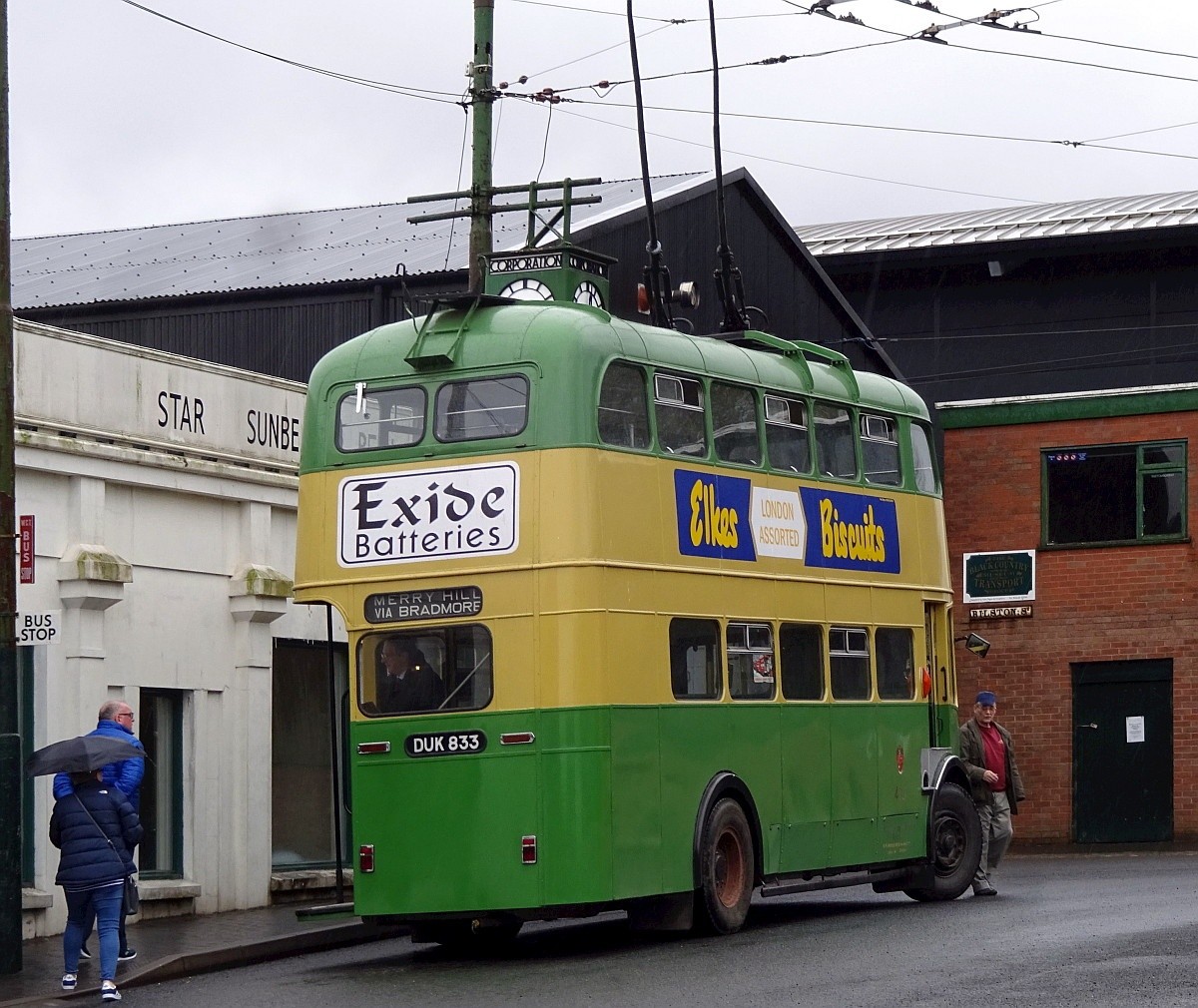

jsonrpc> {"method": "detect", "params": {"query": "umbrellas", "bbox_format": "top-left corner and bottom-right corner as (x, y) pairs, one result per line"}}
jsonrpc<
(27, 737), (146, 777)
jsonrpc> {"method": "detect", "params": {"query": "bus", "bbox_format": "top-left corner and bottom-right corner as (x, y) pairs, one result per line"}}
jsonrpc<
(292, 178), (994, 952)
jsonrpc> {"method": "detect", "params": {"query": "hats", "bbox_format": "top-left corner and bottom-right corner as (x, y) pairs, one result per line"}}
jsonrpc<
(976, 691), (996, 706)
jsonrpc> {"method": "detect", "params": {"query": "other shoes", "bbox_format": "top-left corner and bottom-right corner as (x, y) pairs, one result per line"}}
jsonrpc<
(975, 888), (997, 896)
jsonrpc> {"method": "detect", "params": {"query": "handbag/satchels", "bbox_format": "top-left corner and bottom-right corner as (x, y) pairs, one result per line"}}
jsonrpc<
(123, 875), (139, 916)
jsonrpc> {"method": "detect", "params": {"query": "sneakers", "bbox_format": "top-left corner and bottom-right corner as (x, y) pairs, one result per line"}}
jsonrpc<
(117, 948), (137, 961)
(62, 974), (78, 990)
(79, 946), (91, 958)
(101, 985), (122, 1000)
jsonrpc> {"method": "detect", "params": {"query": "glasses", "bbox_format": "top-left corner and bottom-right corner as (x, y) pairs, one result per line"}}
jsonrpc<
(113, 712), (134, 720)
(380, 652), (400, 659)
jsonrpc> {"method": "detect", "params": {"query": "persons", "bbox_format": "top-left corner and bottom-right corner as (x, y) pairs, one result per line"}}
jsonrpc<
(959, 692), (1026, 896)
(49, 770), (144, 999)
(377, 636), (445, 714)
(52, 700), (145, 961)
